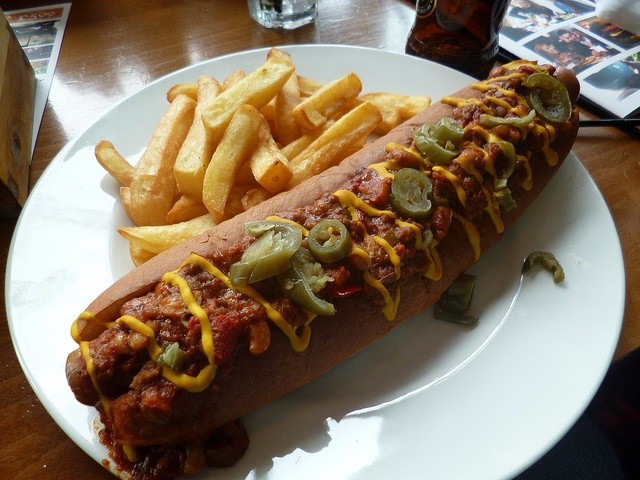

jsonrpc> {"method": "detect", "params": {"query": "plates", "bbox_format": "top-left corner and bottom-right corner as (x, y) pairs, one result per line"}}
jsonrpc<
(4, 43), (627, 480)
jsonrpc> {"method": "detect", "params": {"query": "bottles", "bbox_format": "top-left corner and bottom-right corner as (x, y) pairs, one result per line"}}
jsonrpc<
(405, 0), (512, 85)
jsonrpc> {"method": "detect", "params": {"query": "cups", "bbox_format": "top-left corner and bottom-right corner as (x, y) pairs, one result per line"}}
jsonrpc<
(248, 0), (319, 31)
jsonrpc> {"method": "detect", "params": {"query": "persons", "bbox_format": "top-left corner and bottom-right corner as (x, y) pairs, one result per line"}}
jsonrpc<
(533, 42), (581, 68)
(499, 18), (534, 43)
(558, 30), (620, 57)
(509, 0), (561, 25)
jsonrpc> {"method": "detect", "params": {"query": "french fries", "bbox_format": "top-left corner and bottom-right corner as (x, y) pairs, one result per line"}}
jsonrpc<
(93, 52), (426, 266)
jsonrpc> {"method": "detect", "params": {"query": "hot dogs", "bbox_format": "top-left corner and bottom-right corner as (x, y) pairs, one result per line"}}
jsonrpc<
(63, 57), (581, 480)
